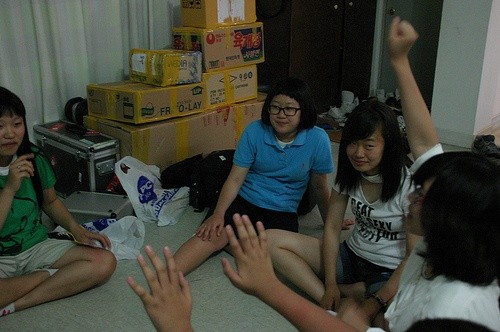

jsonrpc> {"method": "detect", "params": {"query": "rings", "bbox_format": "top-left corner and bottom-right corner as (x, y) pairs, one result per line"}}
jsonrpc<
(17, 165), (21, 168)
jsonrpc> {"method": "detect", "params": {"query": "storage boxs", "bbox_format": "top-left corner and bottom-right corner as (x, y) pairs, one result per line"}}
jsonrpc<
(62, 190), (133, 225)
(172, 22), (265, 73)
(87, 81), (207, 126)
(83, 95), (266, 170)
(180, 0), (256, 29)
(203, 65), (258, 111)
(128, 48), (202, 87)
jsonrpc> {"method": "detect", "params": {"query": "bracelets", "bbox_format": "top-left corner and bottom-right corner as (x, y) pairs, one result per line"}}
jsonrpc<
(373, 293), (388, 308)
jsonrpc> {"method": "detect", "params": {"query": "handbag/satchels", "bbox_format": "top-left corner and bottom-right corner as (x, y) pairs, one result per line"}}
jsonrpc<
(80, 216), (147, 259)
(195, 148), (236, 213)
(114, 158), (190, 227)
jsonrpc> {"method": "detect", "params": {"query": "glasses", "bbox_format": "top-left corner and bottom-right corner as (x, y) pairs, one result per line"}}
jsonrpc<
(413, 185), (425, 203)
(267, 103), (302, 116)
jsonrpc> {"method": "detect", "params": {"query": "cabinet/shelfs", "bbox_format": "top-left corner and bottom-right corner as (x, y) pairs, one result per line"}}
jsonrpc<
(256, 0), (376, 112)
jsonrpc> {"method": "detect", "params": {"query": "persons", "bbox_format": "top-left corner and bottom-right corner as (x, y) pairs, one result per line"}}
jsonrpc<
(173, 83), (355, 278)
(127, 213), (494, 332)
(338, 16), (500, 332)
(265, 98), (422, 324)
(0, 86), (117, 318)
